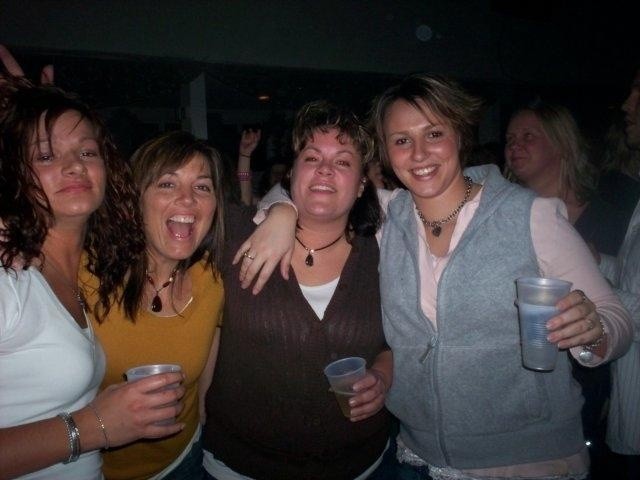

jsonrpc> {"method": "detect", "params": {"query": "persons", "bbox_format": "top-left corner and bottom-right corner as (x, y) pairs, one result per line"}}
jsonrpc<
(2, 42), (639, 480)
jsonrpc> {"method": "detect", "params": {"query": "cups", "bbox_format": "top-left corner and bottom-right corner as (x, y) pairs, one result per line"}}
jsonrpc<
(512, 277), (574, 373)
(125, 364), (182, 426)
(323, 356), (367, 419)
